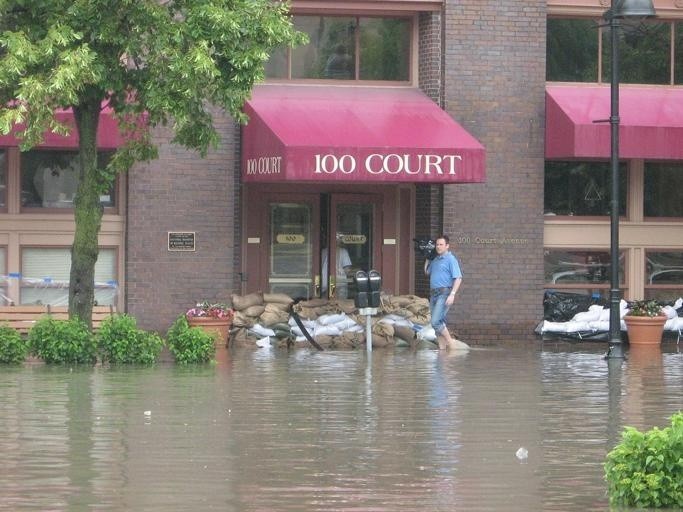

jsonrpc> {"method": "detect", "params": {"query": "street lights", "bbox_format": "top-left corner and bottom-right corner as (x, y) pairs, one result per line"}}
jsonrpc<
(605, 0), (658, 360)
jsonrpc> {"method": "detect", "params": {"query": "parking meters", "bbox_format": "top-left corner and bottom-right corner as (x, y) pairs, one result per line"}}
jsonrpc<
(353, 270), (382, 352)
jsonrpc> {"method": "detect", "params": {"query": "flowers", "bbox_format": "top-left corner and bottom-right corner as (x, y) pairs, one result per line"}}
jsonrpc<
(186, 298), (234, 323)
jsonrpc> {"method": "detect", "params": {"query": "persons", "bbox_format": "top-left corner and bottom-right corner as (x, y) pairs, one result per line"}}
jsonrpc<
(424, 235), (464, 349)
(318, 245), (353, 300)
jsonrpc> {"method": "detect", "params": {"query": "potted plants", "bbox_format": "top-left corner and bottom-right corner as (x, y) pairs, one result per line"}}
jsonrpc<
(622, 298), (668, 345)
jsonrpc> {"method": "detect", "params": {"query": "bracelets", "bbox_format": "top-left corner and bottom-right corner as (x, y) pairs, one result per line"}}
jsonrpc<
(450, 291), (457, 296)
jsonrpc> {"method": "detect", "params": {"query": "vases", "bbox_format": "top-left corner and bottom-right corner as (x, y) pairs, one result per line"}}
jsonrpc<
(187, 317), (233, 349)
